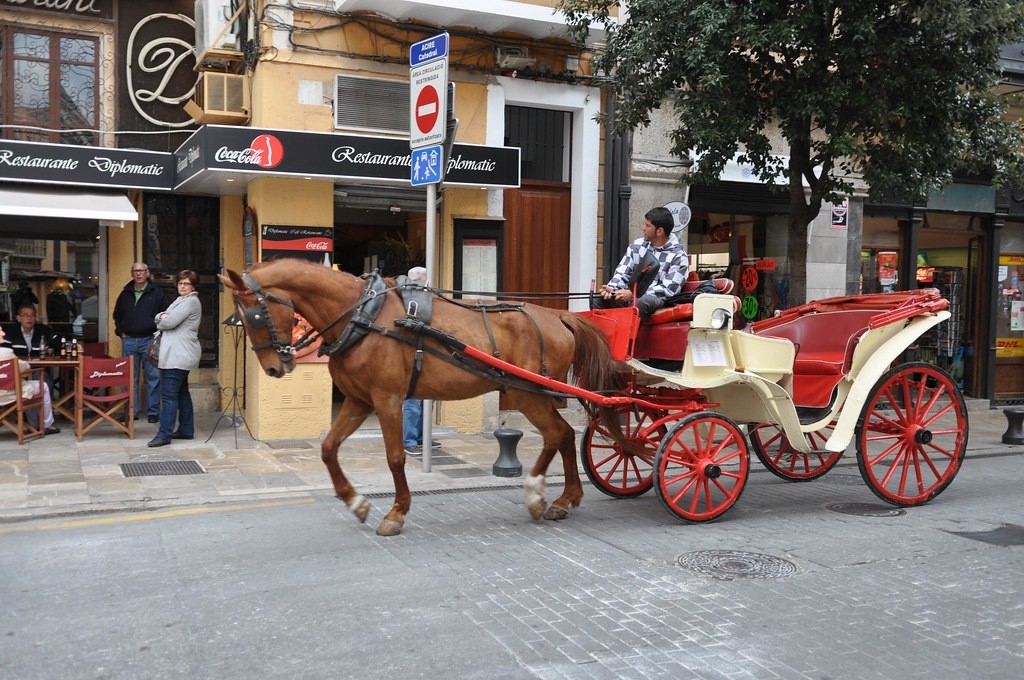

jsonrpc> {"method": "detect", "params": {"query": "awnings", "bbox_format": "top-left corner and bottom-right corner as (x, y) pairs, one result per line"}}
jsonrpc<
(0, 183), (138, 222)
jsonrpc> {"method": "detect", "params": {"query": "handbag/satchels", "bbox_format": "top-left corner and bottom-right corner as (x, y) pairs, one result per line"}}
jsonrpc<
(145, 331), (162, 360)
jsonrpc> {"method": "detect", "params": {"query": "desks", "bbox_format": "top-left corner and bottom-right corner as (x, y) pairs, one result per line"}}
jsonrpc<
(15, 355), (90, 437)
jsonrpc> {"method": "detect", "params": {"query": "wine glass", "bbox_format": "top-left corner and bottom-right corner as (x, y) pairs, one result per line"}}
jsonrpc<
(67, 350), (71, 358)
(47, 349), (54, 357)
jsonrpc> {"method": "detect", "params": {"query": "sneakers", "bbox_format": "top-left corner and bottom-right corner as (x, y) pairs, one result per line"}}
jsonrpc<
(418, 441), (441, 447)
(405, 446), (422, 455)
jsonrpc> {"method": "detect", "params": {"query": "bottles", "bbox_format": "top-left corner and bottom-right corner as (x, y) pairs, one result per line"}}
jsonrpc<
(61, 336), (66, 359)
(40, 336), (45, 360)
(88, 272), (99, 286)
(70, 271), (84, 286)
(71, 336), (78, 359)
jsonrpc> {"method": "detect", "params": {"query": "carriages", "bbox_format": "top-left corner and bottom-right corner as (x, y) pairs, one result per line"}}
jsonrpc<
(215, 257), (971, 536)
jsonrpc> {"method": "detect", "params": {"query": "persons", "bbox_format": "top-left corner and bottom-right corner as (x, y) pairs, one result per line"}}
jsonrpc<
(113, 263), (168, 422)
(593, 207), (689, 318)
(0, 326), (61, 436)
(403, 267), (442, 455)
(47, 286), (77, 322)
(4, 302), (60, 389)
(148, 270), (202, 447)
(11, 281), (39, 321)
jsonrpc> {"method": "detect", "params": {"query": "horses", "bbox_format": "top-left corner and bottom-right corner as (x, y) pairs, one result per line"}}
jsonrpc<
(216, 261), (634, 538)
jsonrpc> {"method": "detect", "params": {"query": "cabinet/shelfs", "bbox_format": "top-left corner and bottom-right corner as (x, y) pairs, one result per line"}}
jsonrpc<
(914, 266), (965, 358)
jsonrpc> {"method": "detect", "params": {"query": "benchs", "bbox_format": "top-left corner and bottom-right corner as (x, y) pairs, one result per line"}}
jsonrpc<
(756, 309), (893, 408)
(633, 278), (735, 360)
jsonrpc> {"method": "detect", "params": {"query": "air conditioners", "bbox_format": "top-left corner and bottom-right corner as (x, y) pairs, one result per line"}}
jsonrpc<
(195, 0), (241, 69)
(194, 71), (248, 124)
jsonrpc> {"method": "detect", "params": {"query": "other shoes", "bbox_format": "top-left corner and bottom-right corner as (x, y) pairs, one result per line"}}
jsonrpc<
(116, 415), (138, 422)
(148, 416), (158, 422)
(44, 427), (60, 435)
(148, 436), (171, 447)
(171, 431), (193, 439)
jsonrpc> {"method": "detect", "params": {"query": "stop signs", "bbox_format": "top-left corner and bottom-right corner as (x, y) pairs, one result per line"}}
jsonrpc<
(408, 58), (445, 148)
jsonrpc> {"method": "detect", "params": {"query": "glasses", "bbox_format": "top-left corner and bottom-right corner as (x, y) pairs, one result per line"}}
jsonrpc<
(132, 270), (147, 273)
(178, 282), (191, 286)
(19, 313), (36, 318)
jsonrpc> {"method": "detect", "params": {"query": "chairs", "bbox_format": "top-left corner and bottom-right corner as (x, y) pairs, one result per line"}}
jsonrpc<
(0, 342), (135, 442)
